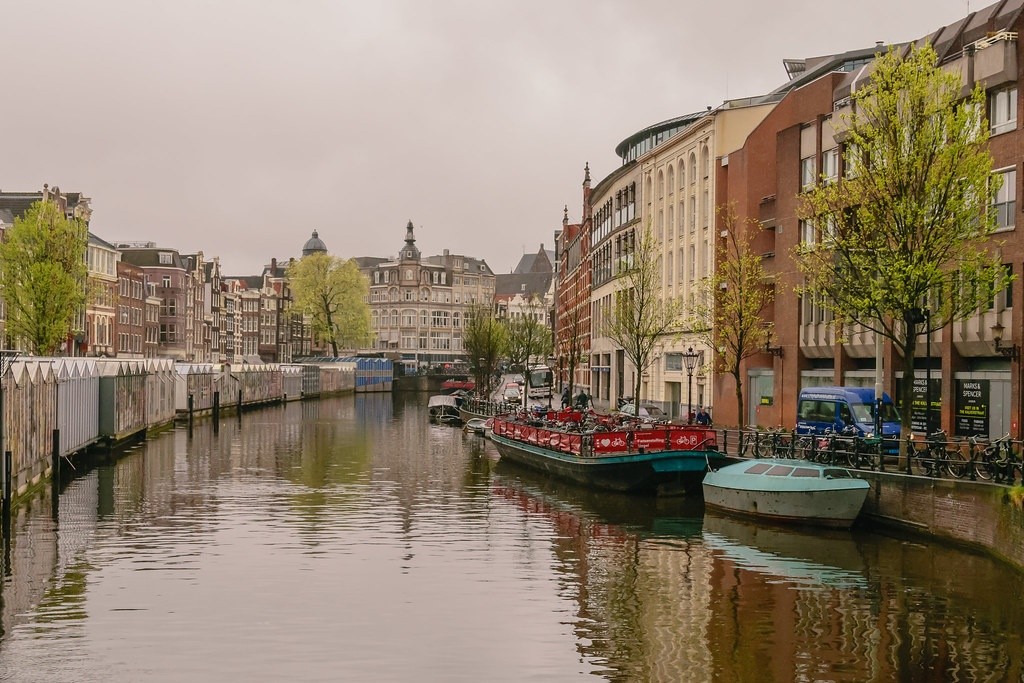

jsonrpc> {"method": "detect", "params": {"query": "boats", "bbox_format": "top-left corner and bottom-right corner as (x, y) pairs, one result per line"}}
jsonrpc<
(428, 389), (466, 423)
(467, 417), (487, 432)
(702, 457), (880, 531)
(481, 417), (494, 438)
(490, 406), (728, 492)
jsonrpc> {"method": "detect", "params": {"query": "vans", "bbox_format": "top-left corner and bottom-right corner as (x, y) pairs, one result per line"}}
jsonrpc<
(796, 387), (915, 461)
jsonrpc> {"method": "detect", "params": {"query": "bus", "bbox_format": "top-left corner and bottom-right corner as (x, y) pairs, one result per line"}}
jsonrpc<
(523, 365), (556, 399)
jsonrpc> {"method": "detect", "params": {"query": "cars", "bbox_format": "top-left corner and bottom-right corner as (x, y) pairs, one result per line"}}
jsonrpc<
(617, 404), (669, 421)
(502, 382), (523, 406)
(512, 374), (526, 385)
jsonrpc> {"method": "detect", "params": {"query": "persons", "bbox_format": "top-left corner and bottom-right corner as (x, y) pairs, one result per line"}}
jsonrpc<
(617, 395), (624, 410)
(560, 388), (569, 409)
(575, 390), (589, 408)
(695, 407), (713, 425)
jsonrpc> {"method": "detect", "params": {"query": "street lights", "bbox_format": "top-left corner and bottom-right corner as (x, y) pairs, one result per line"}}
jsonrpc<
(682, 345), (699, 426)
(905, 308), (931, 437)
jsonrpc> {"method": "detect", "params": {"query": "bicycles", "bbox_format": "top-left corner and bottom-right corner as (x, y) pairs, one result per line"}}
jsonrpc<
(740, 424), (1024, 486)
(512, 402), (662, 452)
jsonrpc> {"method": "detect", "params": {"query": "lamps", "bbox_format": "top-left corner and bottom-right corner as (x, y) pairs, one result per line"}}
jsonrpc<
(989, 322), (1017, 362)
(764, 332), (783, 360)
